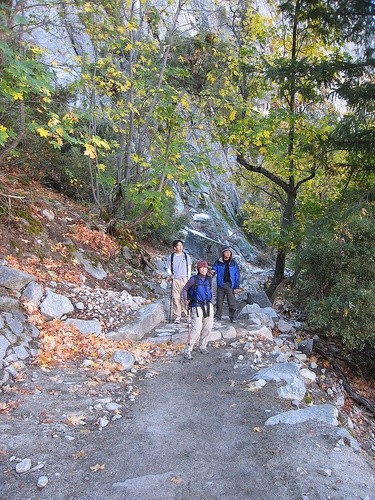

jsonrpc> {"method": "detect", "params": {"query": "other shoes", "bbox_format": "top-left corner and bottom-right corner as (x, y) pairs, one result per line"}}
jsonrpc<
(184, 318), (191, 323)
(184, 353), (193, 360)
(174, 318), (181, 323)
(230, 318), (236, 323)
(198, 349), (209, 354)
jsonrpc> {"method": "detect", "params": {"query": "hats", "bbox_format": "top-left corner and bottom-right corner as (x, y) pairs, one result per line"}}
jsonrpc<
(197, 260), (209, 270)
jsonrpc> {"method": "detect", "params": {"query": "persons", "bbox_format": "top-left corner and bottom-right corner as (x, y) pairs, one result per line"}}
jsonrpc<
(166, 240), (193, 324)
(182, 261), (214, 360)
(208, 246), (240, 323)
